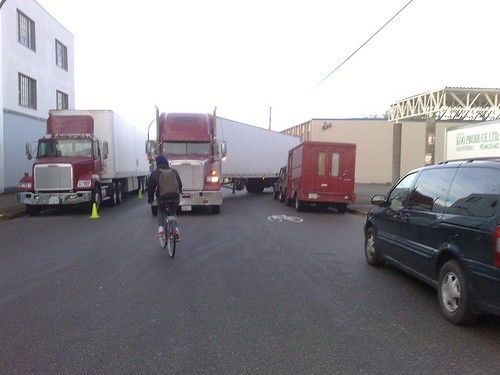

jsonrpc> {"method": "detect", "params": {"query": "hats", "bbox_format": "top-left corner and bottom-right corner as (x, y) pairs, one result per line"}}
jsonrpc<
(157, 156), (168, 166)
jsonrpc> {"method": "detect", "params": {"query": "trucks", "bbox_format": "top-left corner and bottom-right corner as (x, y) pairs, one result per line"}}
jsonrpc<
(284, 140), (357, 212)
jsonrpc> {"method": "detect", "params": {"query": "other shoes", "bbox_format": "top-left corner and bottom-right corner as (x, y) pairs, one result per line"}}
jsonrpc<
(157, 232), (164, 237)
(171, 229), (177, 234)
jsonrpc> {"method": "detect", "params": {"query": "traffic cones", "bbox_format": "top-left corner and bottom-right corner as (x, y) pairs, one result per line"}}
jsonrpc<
(138, 189), (143, 199)
(89, 202), (100, 219)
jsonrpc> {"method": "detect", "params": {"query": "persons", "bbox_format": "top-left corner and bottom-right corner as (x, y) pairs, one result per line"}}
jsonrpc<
(148, 155), (183, 242)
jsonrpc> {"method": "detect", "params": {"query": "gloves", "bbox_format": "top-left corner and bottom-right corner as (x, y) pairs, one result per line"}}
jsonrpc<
(148, 199), (154, 204)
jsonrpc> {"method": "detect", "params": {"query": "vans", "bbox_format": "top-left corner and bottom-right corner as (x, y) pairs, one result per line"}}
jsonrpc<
(363, 158), (500, 325)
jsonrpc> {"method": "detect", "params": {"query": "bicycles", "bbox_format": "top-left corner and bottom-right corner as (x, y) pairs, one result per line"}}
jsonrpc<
(150, 200), (178, 258)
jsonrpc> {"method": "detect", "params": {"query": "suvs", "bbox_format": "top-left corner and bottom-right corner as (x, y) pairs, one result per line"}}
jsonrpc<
(273, 166), (287, 203)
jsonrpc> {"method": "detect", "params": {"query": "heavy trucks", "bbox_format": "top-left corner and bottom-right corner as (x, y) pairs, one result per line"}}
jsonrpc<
(145, 104), (303, 215)
(15, 108), (152, 215)
